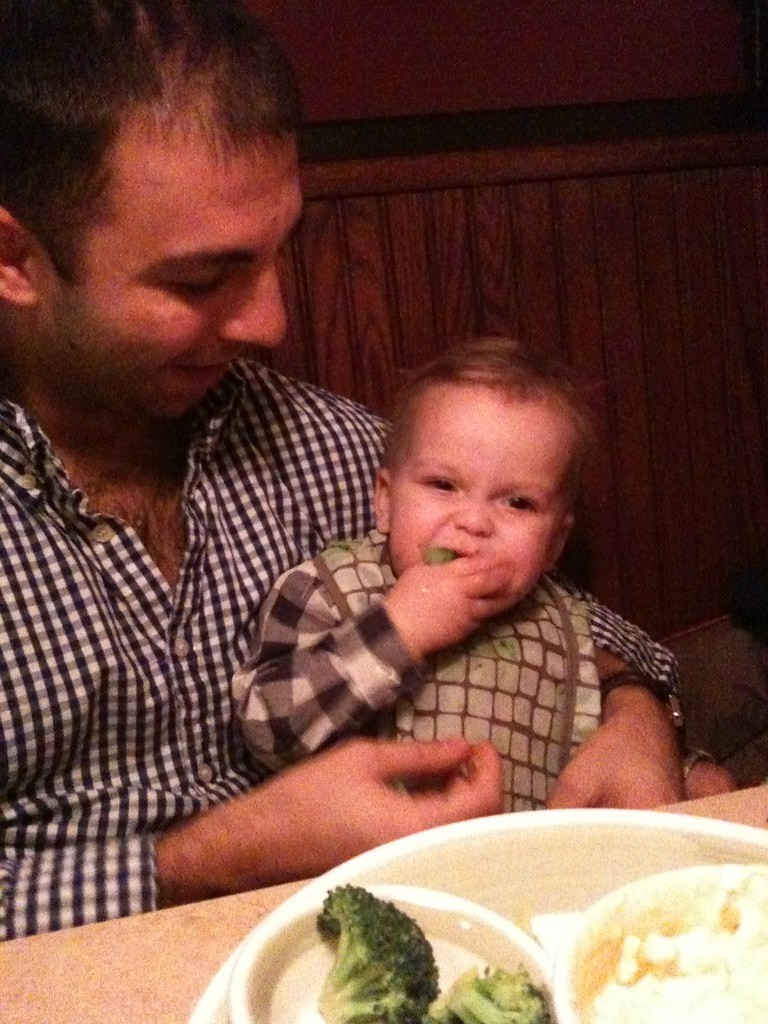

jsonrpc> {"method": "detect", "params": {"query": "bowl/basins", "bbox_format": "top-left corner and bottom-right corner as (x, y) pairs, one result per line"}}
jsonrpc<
(228, 885), (563, 1024)
(554, 863), (768, 1024)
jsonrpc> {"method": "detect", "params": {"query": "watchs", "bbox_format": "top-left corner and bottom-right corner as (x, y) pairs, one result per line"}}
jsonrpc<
(600, 670), (687, 728)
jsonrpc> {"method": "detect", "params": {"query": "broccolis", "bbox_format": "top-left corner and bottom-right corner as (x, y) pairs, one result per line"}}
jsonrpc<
(442, 967), (542, 1024)
(317, 886), (439, 1024)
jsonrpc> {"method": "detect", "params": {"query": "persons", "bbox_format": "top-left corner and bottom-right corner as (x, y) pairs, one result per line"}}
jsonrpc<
(230, 336), (602, 815)
(0, 0), (737, 941)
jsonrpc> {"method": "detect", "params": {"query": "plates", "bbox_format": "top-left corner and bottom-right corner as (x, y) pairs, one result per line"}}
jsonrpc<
(193, 806), (768, 1024)
